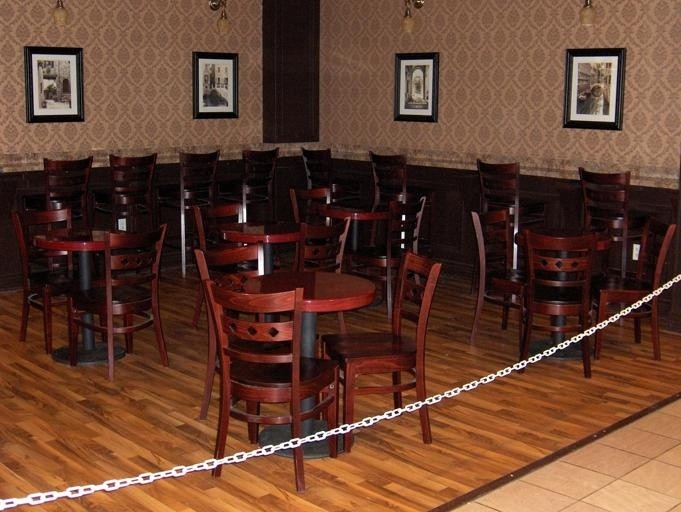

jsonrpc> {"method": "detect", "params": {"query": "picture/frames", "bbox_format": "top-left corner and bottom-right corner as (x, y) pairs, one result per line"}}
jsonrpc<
(191, 51), (239, 119)
(562, 47), (626, 131)
(393, 52), (440, 122)
(24, 45), (84, 124)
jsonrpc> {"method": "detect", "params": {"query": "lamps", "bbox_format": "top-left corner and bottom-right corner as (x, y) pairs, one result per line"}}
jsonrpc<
(400, 0), (425, 34)
(208, 1), (230, 35)
(578, 0), (597, 28)
(52, 0), (68, 28)
